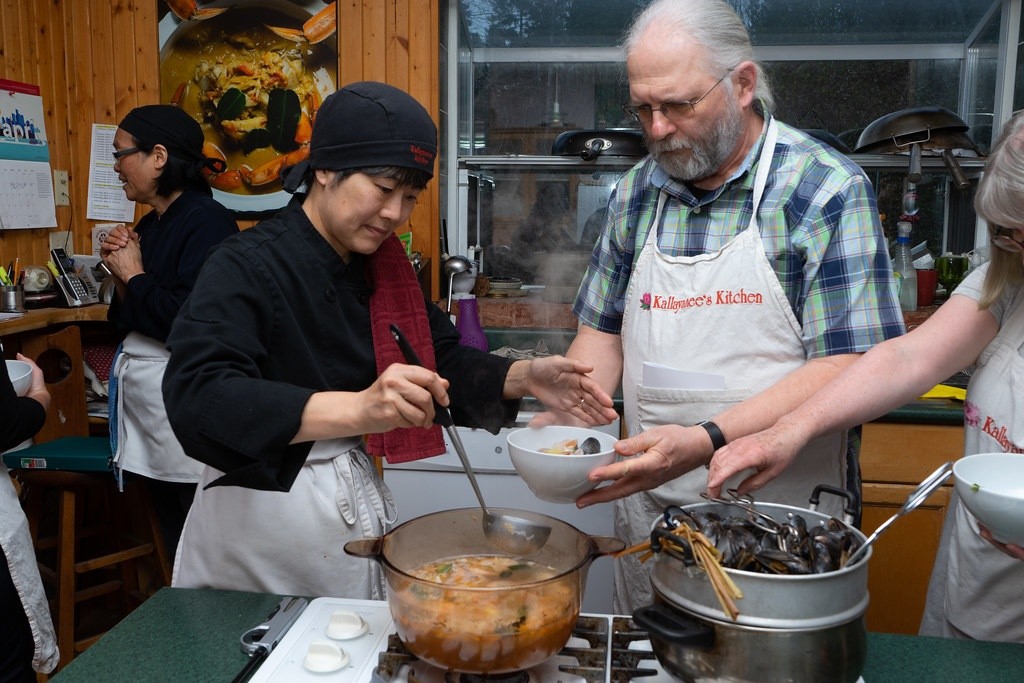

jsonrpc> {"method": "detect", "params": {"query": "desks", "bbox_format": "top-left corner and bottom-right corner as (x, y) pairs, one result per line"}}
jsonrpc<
(0, 300), (109, 682)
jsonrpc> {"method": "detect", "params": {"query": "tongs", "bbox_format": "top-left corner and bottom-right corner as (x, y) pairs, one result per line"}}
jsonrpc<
(700, 489), (798, 552)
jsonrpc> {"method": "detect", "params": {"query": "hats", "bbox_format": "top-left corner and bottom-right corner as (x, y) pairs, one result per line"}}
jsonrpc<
(277, 82), (437, 192)
(119, 102), (227, 174)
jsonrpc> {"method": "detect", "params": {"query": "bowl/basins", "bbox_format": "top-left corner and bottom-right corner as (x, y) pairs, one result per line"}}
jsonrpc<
(952, 453), (1024, 547)
(506, 426), (619, 504)
(4, 360), (33, 398)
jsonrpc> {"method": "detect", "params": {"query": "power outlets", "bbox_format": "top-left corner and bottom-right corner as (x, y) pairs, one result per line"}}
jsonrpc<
(54, 169), (69, 205)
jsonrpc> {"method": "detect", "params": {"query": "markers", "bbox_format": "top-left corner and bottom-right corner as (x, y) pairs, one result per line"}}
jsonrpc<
(0, 267), (13, 286)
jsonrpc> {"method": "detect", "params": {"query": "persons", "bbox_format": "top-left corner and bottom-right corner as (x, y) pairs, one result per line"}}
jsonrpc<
(162, 79), (620, 599)
(705, 111), (1024, 643)
(100, 106), (241, 551)
(524, 0), (904, 616)
(0, 347), (59, 683)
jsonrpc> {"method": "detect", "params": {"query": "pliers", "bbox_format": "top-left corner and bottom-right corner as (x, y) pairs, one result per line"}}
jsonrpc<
(847, 461), (955, 563)
(700, 488), (782, 533)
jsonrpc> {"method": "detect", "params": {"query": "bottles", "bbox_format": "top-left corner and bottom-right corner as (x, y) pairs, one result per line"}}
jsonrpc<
(910, 240), (934, 270)
(456, 300), (488, 354)
(878, 214), (889, 249)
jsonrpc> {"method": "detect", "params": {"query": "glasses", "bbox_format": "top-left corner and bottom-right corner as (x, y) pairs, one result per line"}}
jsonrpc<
(622, 64), (740, 125)
(991, 225), (1023, 253)
(113, 147), (143, 165)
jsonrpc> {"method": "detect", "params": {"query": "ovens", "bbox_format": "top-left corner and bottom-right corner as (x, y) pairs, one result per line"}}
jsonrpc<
(379, 326), (625, 614)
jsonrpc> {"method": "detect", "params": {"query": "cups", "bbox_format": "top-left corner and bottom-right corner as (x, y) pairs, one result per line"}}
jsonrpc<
(0, 284), (25, 313)
(934, 257), (967, 300)
(917, 269), (937, 305)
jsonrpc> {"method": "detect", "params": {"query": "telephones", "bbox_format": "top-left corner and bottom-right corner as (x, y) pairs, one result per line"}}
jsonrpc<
(51, 247), (99, 309)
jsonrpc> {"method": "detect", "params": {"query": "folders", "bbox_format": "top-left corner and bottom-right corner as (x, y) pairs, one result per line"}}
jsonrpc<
(2, 436), (113, 471)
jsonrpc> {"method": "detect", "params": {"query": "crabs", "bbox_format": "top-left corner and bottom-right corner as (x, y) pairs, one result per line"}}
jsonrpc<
(164, 0), (338, 192)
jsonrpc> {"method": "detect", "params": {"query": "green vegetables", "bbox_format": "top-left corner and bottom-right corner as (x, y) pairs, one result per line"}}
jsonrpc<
(217, 87), (301, 153)
(434, 563), (529, 616)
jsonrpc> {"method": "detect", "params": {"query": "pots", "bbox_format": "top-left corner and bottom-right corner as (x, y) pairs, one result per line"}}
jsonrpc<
(837, 127), (865, 150)
(796, 129), (852, 153)
(344, 507), (627, 674)
(855, 105), (979, 190)
(633, 483), (873, 683)
(552, 126), (647, 161)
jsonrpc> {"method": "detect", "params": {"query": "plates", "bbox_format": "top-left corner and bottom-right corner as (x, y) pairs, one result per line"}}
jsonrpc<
(158, 0), (334, 212)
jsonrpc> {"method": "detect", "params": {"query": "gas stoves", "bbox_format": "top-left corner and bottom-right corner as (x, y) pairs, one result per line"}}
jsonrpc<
(247, 598), (866, 683)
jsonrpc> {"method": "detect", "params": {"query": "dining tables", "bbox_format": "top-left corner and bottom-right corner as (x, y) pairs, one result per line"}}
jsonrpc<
(48, 585), (1024, 682)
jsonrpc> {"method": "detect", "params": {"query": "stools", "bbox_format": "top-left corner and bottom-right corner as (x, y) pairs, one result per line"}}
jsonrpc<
(4, 438), (171, 671)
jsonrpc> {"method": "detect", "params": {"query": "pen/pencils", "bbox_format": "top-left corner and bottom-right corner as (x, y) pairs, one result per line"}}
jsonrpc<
(0, 258), (25, 287)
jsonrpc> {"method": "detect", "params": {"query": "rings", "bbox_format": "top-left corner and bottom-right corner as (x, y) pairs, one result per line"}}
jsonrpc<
(580, 398), (585, 408)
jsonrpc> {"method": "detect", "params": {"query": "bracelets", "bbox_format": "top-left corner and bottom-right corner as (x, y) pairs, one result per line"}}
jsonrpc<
(697, 420), (725, 451)
